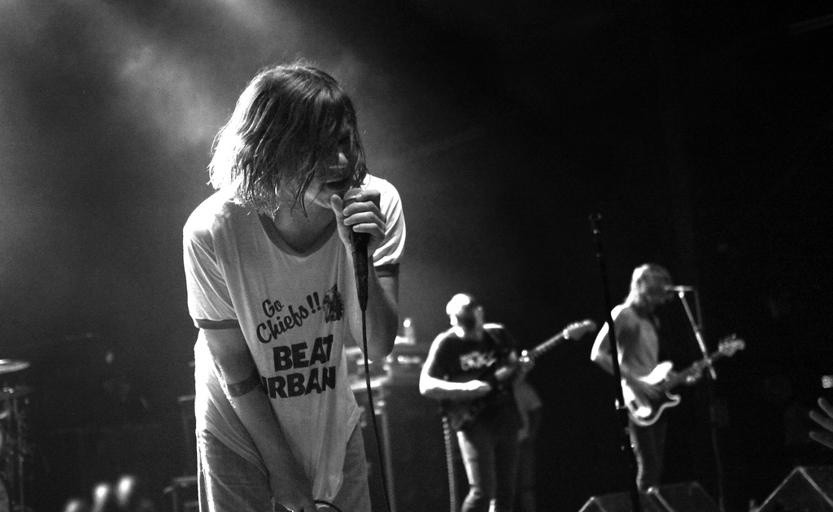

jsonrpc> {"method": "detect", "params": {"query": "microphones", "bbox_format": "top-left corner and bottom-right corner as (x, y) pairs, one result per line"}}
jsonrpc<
(341, 187), (380, 312)
(659, 283), (698, 299)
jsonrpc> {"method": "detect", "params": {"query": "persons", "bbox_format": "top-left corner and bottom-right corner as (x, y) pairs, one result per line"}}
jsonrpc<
(590, 263), (703, 489)
(183, 62), (406, 512)
(809, 398), (833, 449)
(418, 292), (535, 512)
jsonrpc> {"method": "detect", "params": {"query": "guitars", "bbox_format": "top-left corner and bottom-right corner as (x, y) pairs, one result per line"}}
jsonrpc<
(439, 320), (597, 431)
(621, 335), (746, 428)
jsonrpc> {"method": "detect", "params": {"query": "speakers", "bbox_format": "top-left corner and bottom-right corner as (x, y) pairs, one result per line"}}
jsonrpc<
(577, 491), (670, 512)
(757, 465), (832, 512)
(648, 479), (719, 512)
